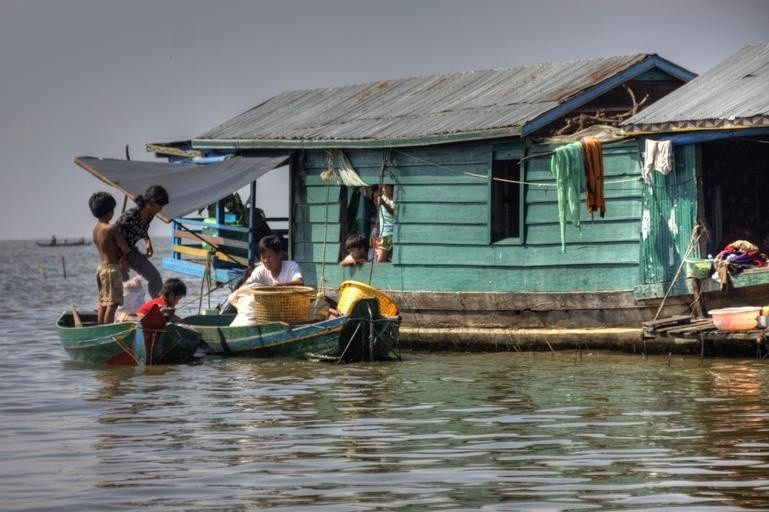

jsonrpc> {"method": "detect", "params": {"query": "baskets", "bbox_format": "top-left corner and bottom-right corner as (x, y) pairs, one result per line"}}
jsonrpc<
(247, 286), (316, 323)
(335, 280), (403, 318)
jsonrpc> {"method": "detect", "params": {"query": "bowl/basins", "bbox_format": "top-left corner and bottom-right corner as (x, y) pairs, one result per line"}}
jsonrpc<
(707, 306), (762, 333)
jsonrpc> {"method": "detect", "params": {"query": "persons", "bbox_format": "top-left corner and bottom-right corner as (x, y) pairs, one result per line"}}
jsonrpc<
(369, 185), (394, 263)
(240, 234), (305, 293)
(94, 184), (170, 312)
(89, 191), (130, 325)
(338, 232), (377, 266)
(135, 277), (187, 355)
(108, 260), (147, 322)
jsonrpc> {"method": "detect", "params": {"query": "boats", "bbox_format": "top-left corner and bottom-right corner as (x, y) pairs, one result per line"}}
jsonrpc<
(56, 307), (203, 367)
(35, 237), (94, 247)
(182, 297), (402, 364)
